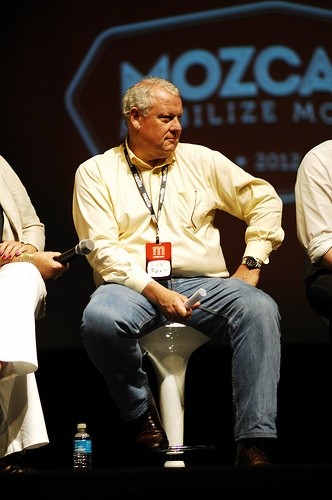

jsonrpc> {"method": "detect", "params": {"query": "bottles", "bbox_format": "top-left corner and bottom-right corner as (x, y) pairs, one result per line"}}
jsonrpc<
(73, 422), (91, 470)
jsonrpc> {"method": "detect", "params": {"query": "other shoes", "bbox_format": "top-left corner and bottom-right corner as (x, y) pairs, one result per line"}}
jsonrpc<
(0, 463), (32, 475)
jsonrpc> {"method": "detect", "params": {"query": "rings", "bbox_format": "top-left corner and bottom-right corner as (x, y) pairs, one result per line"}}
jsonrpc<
(54, 274), (59, 280)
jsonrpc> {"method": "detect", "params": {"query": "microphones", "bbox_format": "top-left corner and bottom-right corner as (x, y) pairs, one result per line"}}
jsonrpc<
(54, 239), (95, 264)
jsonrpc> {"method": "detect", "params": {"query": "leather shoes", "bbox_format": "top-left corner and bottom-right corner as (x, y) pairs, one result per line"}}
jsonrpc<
(135, 388), (168, 451)
(235, 446), (272, 470)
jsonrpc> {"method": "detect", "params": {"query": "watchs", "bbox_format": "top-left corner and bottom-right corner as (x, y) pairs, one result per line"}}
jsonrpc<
(241, 256), (261, 269)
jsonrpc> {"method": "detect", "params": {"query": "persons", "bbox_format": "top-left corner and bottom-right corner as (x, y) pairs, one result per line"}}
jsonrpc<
(72, 77), (286, 466)
(294, 136), (332, 322)
(0, 153), (70, 475)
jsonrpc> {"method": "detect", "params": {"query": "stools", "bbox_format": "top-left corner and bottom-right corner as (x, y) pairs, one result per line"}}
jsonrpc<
(141, 322), (213, 468)
